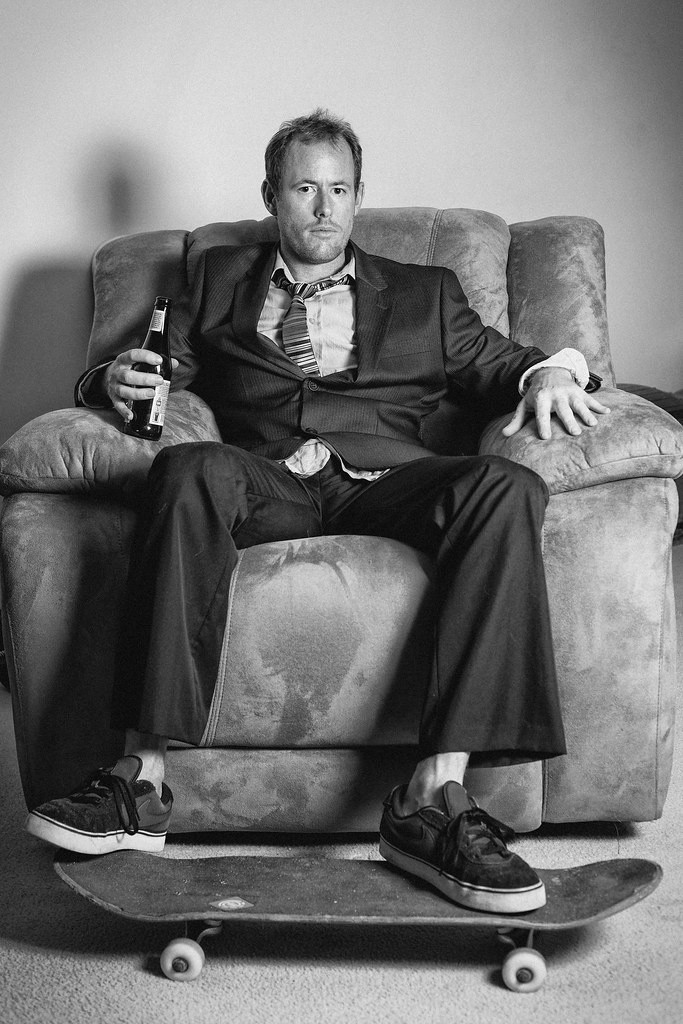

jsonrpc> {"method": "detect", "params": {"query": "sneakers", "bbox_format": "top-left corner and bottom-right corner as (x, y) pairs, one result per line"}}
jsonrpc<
(380, 781), (547, 913)
(24, 755), (174, 855)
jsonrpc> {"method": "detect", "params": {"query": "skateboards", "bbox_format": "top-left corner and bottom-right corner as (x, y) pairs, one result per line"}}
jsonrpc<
(51, 849), (664, 995)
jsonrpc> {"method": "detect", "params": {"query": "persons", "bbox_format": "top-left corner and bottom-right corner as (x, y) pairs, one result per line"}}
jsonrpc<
(23, 108), (611, 913)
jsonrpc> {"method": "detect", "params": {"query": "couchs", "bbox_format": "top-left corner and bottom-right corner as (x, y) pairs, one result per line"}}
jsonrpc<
(0, 206), (683, 842)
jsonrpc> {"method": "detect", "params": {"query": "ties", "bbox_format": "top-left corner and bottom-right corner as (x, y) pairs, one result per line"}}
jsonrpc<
(271, 269), (353, 377)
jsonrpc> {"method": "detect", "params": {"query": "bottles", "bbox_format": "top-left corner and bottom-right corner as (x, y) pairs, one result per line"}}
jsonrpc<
(124, 296), (173, 441)
